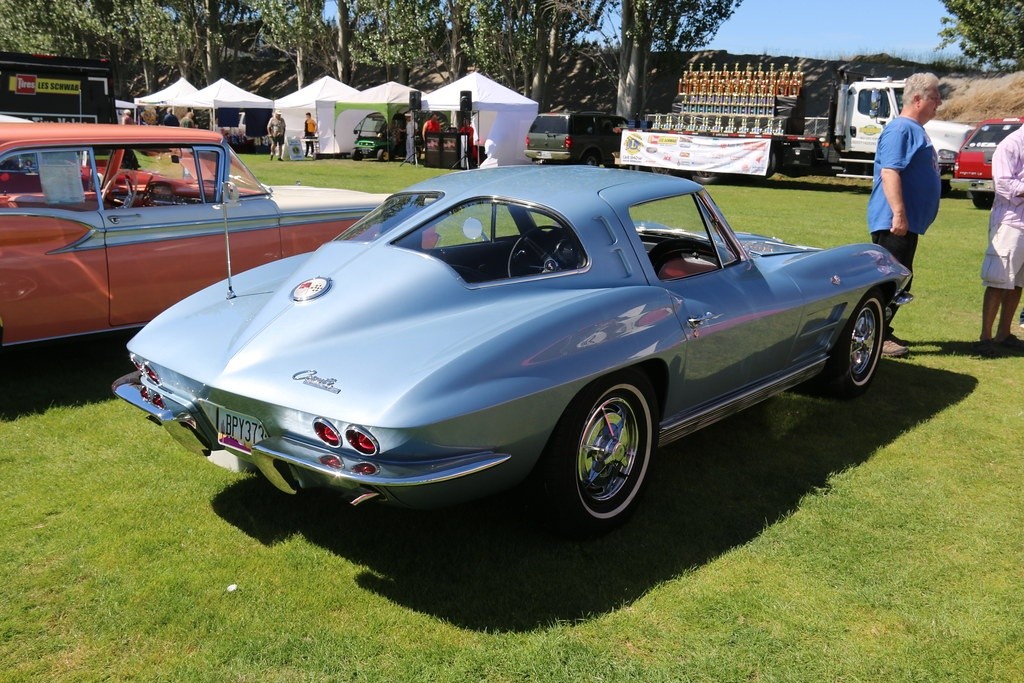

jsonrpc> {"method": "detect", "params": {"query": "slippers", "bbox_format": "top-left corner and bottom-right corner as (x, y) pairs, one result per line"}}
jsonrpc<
(995, 334), (1024, 351)
(979, 339), (999, 357)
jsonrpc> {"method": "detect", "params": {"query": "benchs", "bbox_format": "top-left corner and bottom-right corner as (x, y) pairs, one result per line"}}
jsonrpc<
(0, 192), (113, 212)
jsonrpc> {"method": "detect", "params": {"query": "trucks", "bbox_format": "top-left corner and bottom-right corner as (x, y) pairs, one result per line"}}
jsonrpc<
(617, 76), (976, 186)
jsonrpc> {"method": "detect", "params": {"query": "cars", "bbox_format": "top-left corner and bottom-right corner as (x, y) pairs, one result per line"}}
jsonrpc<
(110, 166), (914, 529)
(0, 122), (393, 356)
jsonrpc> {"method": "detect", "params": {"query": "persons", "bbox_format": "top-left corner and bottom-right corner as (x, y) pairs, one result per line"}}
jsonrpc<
(164, 108), (180, 127)
(181, 112), (194, 128)
(267, 110), (286, 161)
(970, 122), (1024, 358)
(422, 113), (441, 141)
(400, 113), (418, 165)
(304, 113), (318, 157)
(122, 109), (132, 125)
(867, 72), (941, 356)
(460, 117), (474, 155)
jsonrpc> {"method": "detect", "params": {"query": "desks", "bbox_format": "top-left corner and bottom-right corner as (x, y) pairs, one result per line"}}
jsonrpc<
(280, 138), (319, 161)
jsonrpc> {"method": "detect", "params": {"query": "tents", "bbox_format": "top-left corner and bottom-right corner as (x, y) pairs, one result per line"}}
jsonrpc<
(115, 100), (140, 125)
(134, 77), (198, 125)
(415, 71), (539, 169)
(274, 75), (428, 163)
(168, 77), (274, 154)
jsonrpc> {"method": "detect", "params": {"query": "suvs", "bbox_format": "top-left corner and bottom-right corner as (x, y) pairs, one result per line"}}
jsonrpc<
(350, 112), (407, 161)
(950, 117), (1024, 210)
(523, 109), (629, 165)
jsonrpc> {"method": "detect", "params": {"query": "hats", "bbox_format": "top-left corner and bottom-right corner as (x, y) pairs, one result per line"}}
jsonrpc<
(405, 114), (412, 118)
(275, 110), (282, 114)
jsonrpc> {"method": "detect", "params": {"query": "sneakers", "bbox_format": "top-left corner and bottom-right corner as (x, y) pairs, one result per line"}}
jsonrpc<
(881, 334), (909, 356)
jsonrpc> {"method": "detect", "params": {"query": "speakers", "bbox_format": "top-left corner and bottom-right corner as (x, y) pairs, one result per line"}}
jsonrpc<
(461, 90), (472, 111)
(409, 90), (421, 110)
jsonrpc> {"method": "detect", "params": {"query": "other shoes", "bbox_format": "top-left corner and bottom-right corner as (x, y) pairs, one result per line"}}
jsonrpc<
(271, 155), (273, 160)
(404, 160), (410, 163)
(1020, 324), (1024, 328)
(278, 158), (283, 161)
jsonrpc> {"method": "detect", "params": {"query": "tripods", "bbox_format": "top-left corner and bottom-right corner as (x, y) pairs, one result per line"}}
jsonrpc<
(400, 110), (422, 167)
(452, 111), (478, 170)
